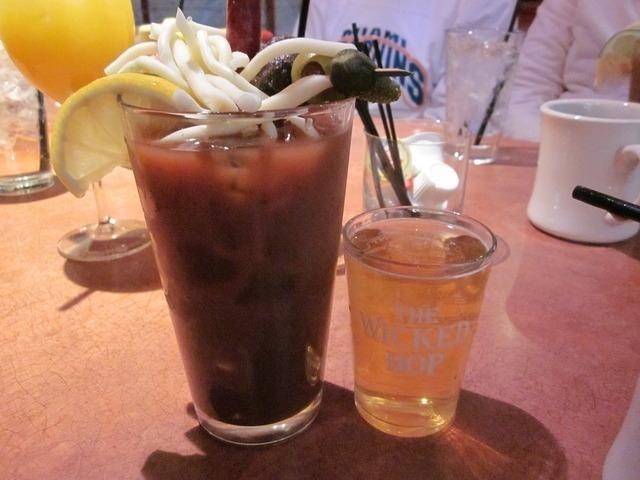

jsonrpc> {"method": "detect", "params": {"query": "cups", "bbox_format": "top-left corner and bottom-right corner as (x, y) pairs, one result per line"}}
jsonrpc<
(340, 206), (498, 444)
(113, 92), (360, 442)
(444, 27), (524, 164)
(528, 97), (640, 243)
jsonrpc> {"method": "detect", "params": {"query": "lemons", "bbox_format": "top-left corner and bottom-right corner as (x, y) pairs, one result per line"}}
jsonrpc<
(49, 72), (196, 197)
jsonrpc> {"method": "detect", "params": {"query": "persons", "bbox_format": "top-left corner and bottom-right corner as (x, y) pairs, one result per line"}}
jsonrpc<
(290, 1), (640, 143)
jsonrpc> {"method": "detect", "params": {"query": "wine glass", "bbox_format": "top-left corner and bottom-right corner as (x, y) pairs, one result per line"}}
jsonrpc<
(0, 1), (151, 263)
